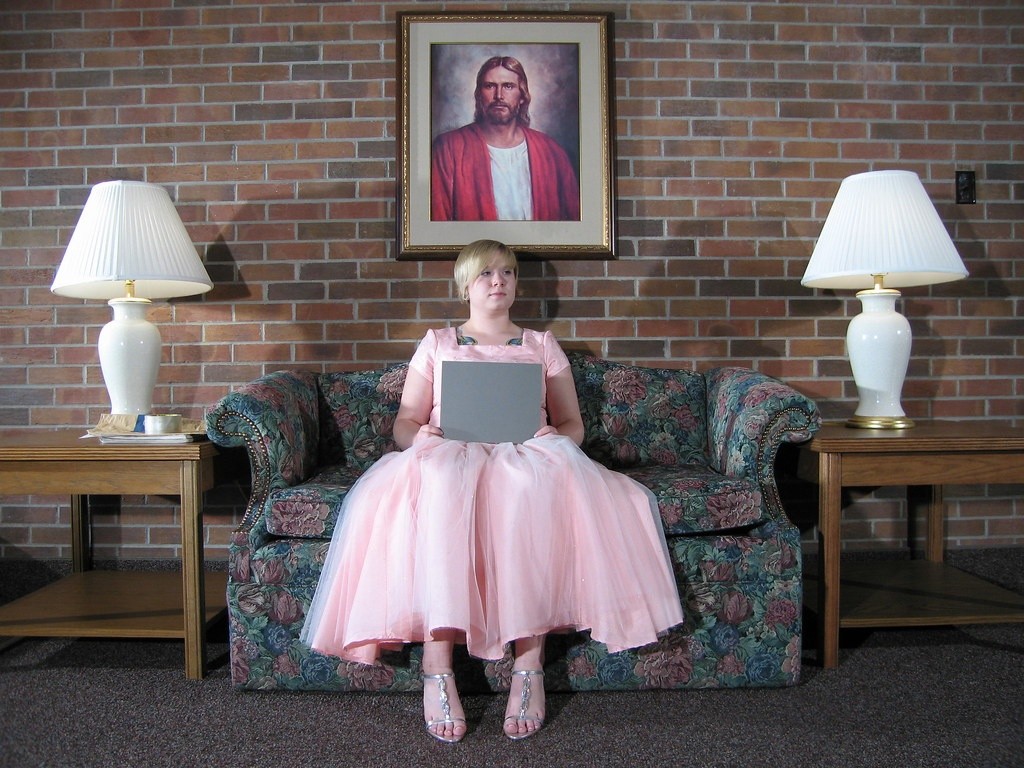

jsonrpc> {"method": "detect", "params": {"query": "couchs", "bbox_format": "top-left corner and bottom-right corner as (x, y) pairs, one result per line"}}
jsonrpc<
(202, 352), (825, 692)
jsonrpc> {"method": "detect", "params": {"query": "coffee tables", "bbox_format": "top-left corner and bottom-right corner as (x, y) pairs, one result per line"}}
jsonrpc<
(773, 419), (1024, 670)
(0, 429), (254, 680)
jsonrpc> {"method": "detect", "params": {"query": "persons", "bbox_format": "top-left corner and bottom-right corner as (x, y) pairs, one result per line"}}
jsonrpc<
(297, 240), (686, 742)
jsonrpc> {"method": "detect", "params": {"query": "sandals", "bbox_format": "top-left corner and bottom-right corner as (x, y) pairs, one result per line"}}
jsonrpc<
(421, 672), (467, 743)
(503, 670), (545, 740)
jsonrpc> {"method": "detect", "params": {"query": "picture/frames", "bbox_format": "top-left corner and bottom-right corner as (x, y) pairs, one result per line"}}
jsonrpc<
(393, 9), (618, 261)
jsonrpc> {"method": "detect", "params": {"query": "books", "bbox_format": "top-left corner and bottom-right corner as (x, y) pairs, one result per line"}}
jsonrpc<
(98, 431), (207, 444)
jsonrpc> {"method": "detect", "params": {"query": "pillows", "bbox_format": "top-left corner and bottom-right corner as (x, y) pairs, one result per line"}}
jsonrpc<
(564, 349), (711, 470)
(314, 359), (410, 478)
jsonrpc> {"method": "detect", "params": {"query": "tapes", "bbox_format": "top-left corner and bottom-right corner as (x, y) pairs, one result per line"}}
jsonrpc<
(145, 413), (182, 434)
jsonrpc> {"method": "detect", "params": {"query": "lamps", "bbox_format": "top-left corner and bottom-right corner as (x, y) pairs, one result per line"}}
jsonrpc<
(50, 177), (214, 415)
(799, 169), (972, 431)
